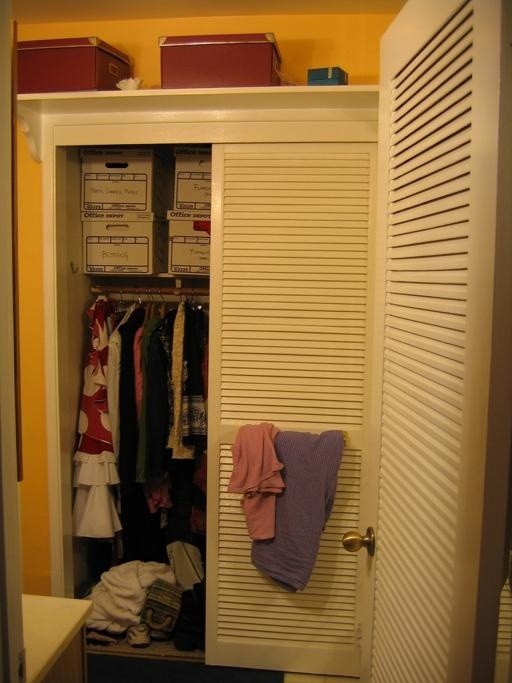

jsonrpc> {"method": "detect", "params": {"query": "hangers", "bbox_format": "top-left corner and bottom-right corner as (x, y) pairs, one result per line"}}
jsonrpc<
(86, 282), (206, 323)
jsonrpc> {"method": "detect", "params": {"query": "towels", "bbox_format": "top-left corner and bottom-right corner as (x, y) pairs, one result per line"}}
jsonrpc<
(251, 428), (344, 595)
(227, 422), (286, 541)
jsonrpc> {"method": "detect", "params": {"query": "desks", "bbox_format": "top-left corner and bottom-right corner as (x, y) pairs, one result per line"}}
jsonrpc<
(19, 595), (95, 681)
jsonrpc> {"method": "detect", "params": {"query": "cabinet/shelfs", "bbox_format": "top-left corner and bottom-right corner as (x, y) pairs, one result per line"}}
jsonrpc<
(14, 85), (379, 678)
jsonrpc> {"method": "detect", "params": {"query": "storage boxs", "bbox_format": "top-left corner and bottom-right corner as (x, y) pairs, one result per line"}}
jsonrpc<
(16, 36), (130, 94)
(77, 143), (212, 276)
(158, 32), (282, 90)
